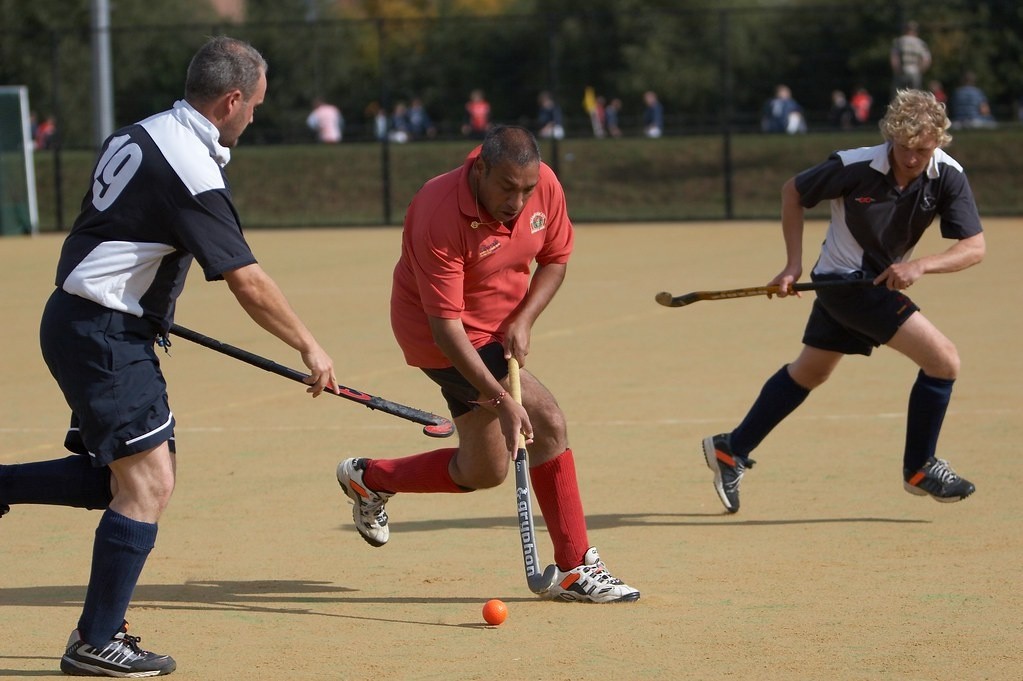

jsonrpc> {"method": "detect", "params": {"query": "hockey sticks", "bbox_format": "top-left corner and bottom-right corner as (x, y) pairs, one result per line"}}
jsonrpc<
(507, 343), (558, 597)
(655, 274), (892, 310)
(167, 319), (456, 439)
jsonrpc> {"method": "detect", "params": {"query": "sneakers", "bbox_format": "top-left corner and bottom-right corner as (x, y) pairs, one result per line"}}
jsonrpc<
(702, 433), (756, 513)
(903, 457), (975, 503)
(542, 546), (640, 603)
(337, 456), (396, 547)
(60, 619), (177, 678)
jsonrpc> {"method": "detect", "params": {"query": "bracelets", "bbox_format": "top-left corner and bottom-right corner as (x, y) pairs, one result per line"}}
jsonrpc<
(467, 392), (509, 410)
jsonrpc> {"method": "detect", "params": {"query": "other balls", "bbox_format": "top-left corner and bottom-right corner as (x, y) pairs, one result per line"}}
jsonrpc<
(483, 599), (508, 626)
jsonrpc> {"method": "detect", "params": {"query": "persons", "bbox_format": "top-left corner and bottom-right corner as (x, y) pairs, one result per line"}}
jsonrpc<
(375, 99), (438, 142)
(460, 90), (492, 139)
(337, 126), (639, 603)
(596, 90), (664, 137)
(307, 96), (343, 143)
(891, 20), (990, 125)
(539, 91), (563, 140)
(701, 89), (984, 512)
(30, 109), (56, 150)
(0, 35), (340, 678)
(762, 85), (873, 133)
